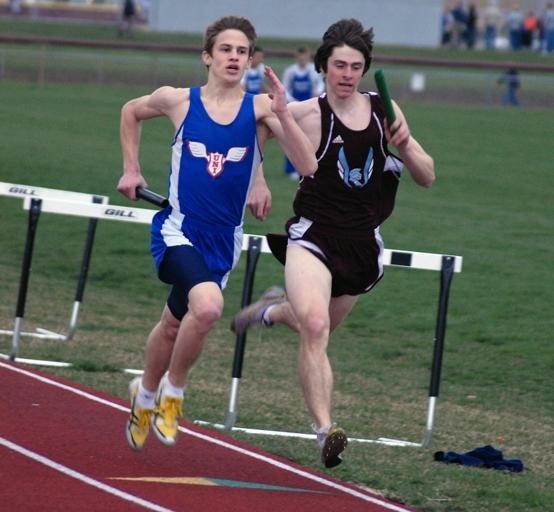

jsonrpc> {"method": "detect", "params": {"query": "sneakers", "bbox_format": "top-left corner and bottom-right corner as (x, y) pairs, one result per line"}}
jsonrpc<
(316, 423), (348, 471)
(230, 284), (287, 334)
(124, 375), (185, 451)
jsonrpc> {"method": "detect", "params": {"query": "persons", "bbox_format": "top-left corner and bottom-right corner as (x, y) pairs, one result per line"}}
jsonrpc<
(115, 15), (319, 454)
(441, 0), (554, 55)
(495, 66), (522, 106)
(240, 46), (267, 94)
(229, 13), (437, 470)
(280, 43), (326, 182)
(118, 0), (137, 41)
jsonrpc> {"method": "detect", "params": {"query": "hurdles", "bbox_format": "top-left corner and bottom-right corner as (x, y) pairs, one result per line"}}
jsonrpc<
(0, 195), (162, 375)
(193, 234), (463, 448)
(0, 182), (111, 343)
